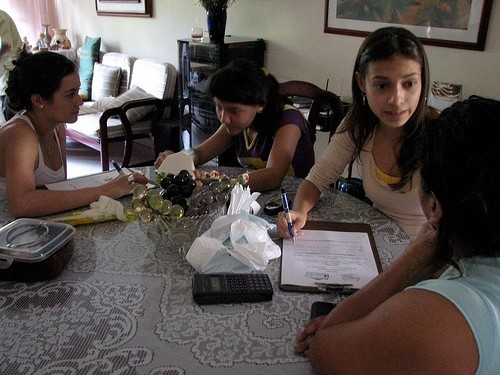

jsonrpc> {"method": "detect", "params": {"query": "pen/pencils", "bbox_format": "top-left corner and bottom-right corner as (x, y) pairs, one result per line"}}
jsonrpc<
(112, 160), (126, 175)
(281, 188), (296, 245)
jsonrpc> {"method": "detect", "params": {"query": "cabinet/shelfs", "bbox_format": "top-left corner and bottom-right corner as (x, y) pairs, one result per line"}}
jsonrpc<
(178, 36), (266, 165)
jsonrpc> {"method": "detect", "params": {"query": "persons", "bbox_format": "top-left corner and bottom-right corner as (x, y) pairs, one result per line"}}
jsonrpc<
(0, 9), (29, 125)
(310, 94), (499, 375)
(154, 59), (316, 191)
(0, 44), (148, 220)
(276, 27), (442, 355)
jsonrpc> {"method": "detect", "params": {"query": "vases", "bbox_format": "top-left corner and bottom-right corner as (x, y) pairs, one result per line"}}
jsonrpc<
(50, 28), (71, 49)
(207, 9), (227, 42)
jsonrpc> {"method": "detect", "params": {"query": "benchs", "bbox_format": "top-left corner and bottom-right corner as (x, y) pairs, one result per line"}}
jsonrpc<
(63, 46), (179, 172)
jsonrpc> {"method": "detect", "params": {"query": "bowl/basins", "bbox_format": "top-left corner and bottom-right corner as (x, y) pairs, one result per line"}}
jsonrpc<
(138, 203), (227, 246)
(0, 218), (76, 281)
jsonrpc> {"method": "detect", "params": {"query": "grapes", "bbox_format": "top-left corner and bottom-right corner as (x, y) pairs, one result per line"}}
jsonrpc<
(129, 170), (231, 224)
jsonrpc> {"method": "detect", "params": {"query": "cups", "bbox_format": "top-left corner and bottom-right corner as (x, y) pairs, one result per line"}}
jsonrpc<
(191, 28), (204, 41)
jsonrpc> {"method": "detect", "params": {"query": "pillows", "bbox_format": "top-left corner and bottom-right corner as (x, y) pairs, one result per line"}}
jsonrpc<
(91, 61), (122, 100)
(91, 97), (116, 112)
(104, 86), (160, 124)
(78, 36), (101, 99)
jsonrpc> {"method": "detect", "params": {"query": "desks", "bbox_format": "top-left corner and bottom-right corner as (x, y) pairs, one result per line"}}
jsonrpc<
(0, 164), (413, 375)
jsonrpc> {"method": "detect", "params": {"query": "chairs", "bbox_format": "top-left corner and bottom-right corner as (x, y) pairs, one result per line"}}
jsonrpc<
(279, 81), (344, 188)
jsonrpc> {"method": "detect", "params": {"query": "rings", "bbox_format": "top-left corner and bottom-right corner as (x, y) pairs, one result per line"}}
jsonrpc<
(129, 175), (135, 182)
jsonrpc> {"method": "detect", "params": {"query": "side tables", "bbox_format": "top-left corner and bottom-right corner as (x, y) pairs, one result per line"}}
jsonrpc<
(31, 47), (77, 61)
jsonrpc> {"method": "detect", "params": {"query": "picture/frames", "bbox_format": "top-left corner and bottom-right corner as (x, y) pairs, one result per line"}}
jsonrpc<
(323, 0), (494, 52)
(95, 0), (152, 17)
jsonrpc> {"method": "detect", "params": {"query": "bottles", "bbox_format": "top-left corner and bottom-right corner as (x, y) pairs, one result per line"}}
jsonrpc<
(49, 29), (71, 50)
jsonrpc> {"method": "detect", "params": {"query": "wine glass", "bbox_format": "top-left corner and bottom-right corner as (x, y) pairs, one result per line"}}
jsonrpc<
(41, 24), (52, 47)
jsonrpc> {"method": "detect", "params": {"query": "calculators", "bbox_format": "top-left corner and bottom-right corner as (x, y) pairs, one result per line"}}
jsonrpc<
(192, 272), (274, 305)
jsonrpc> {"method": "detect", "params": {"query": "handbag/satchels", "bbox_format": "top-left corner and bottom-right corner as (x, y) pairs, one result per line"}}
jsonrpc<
(185, 211), (281, 274)
